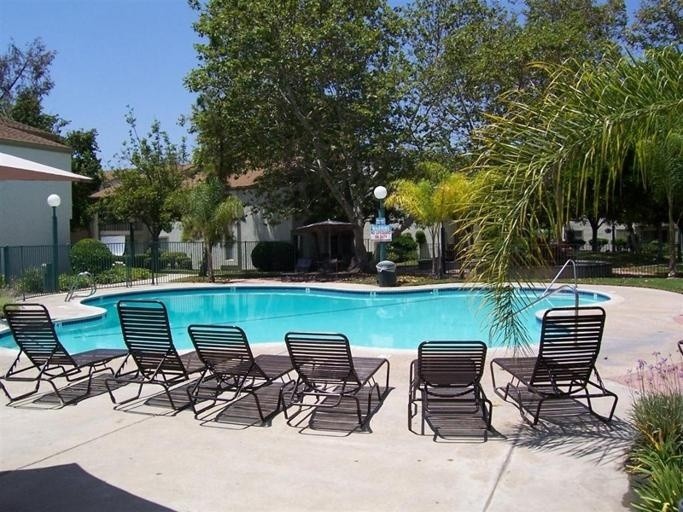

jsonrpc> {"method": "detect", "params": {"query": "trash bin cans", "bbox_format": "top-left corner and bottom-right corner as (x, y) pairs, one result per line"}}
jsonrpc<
(377, 261), (397, 287)
(40, 263), (58, 290)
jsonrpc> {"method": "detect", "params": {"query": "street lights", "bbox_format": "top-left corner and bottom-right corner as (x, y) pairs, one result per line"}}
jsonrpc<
(374, 186), (388, 262)
(47, 193), (61, 292)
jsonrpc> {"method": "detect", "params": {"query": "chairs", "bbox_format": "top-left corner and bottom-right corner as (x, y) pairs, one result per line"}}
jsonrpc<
(187, 323), (316, 430)
(283, 331), (391, 431)
(490, 306), (618, 430)
(105, 299), (243, 419)
(407, 339), (509, 444)
(1, 298), (132, 411)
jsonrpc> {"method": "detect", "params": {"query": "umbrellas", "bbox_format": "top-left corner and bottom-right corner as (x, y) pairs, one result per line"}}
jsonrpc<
(296, 219), (354, 261)
